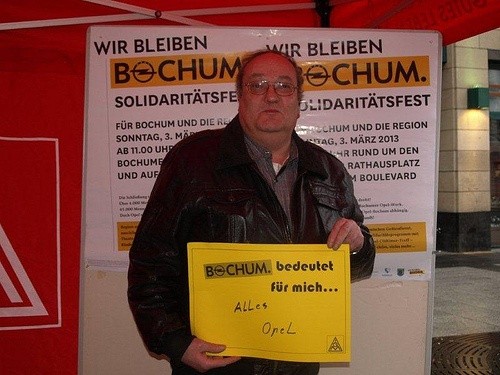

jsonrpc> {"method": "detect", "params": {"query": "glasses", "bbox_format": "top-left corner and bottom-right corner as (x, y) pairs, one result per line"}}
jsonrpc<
(239, 79), (300, 97)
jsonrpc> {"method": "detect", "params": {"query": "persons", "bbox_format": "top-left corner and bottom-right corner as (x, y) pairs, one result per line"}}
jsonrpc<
(126, 49), (376, 375)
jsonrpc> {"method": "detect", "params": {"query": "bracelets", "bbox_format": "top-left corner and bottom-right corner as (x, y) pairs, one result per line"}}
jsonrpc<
(349, 250), (360, 256)
